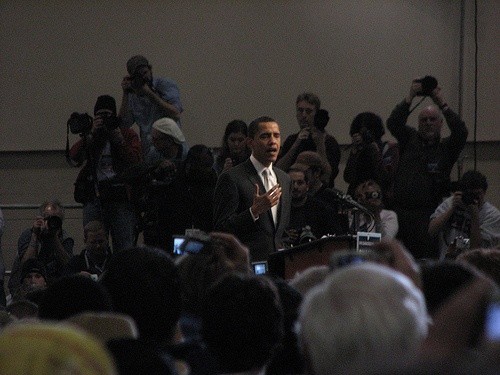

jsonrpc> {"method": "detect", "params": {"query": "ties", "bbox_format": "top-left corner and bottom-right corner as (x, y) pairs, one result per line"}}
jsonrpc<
(262, 169), (271, 190)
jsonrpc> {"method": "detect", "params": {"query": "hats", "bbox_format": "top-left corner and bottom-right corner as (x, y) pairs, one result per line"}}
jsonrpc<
(94, 95), (116, 118)
(127, 56), (148, 75)
(152, 118), (186, 144)
(289, 151), (321, 171)
(20, 259), (49, 287)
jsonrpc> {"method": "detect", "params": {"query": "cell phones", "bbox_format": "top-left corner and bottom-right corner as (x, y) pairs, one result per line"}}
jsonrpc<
(252, 261), (269, 277)
(180, 235), (206, 256)
(172, 235), (187, 258)
(90, 274), (99, 282)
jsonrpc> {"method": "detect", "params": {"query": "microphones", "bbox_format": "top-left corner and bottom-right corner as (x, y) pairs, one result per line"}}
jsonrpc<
(323, 187), (375, 232)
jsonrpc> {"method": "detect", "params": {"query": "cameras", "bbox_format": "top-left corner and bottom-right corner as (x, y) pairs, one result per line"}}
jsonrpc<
(134, 73), (148, 87)
(103, 115), (119, 130)
(460, 191), (479, 205)
(46, 216), (62, 232)
(313, 109), (330, 132)
(362, 130), (376, 143)
(148, 178), (162, 192)
(415, 75), (438, 97)
(365, 192), (380, 200)
(293, 228), (316, 245)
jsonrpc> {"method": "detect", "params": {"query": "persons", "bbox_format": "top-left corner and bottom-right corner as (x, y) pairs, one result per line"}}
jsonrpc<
(68, 76), (470, 260)
(113, 55), (190, 162)
(210, 115), (296, 255)
(425, 171), (500, 261)
(0, 200), (500, 375)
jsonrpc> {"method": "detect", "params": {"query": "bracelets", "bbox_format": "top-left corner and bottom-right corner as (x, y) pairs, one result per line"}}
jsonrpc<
(439, 103), (448, 110)
